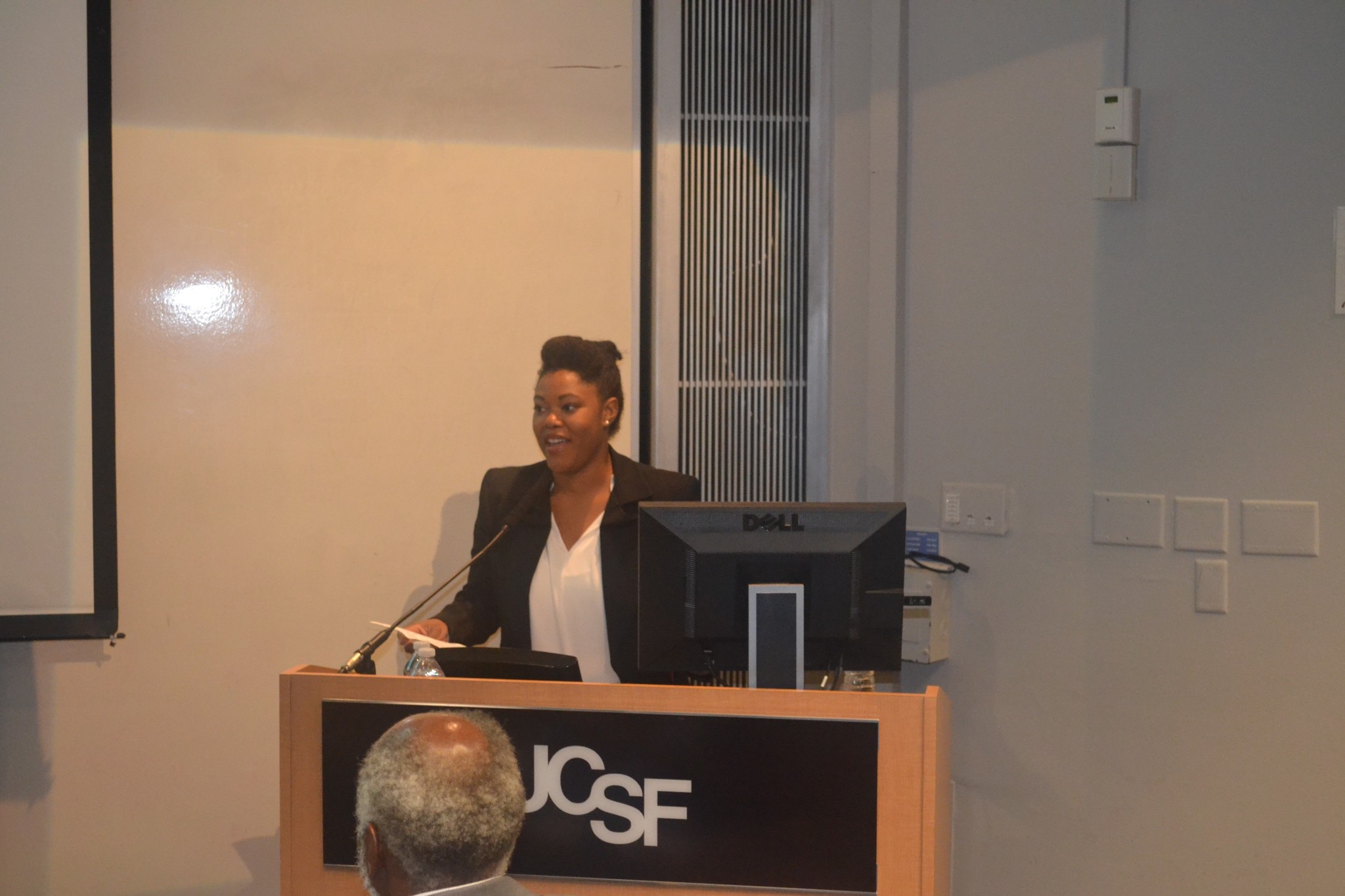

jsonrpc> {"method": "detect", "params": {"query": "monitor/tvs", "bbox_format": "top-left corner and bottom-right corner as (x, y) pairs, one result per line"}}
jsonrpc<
(637, 501), (907, 687)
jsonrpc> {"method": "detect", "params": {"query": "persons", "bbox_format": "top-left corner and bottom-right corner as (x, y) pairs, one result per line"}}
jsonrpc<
(354, 712), (541, 896)
(398, 336), (702, 683)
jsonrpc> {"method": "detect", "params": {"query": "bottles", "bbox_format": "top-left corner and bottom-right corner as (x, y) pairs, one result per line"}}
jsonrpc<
(404, 641), (431, 676)
(406, 648), (445, 676)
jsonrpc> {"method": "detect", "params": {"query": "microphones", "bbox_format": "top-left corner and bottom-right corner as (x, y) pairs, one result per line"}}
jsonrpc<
(338, 493), (525, 673)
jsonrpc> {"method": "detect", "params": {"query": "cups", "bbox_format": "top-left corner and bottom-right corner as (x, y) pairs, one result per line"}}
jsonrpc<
(843, 669), (875, 693)
